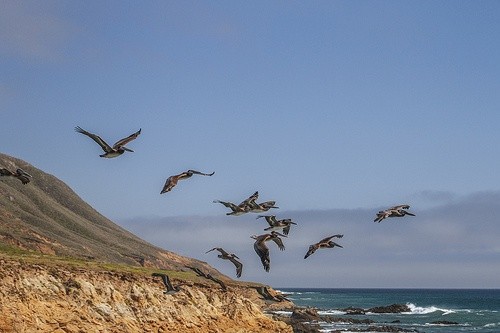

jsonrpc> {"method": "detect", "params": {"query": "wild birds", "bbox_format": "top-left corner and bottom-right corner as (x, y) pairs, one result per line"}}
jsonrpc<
(255, 214), (297, 235)
(250, 234), (286, 273)
(373, 203), (416, 224)
(73, 125), (142, 159)
(159, 169), (217, 196)
(204, 247), (243, 279)
(271, 231), (288, 238)
(303, 233), (345, 260)
(150, 272), (190, 295)
(212, 190), (280, 217)
